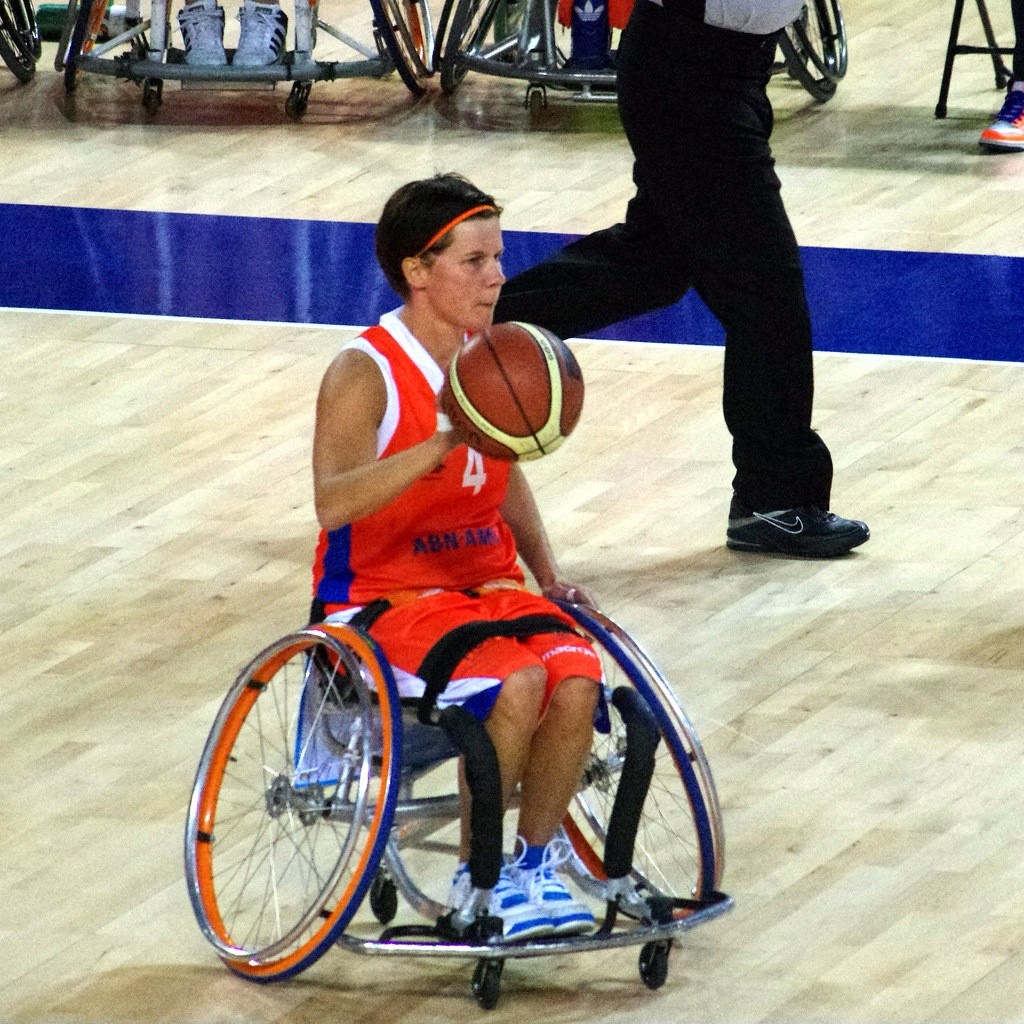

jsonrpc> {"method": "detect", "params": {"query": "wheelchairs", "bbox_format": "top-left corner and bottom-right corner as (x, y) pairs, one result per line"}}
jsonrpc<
(184, 602), (734, 1009)
(54, 1), (435, 120)
(0, 0), (42, 82)
(431, 0), (849, 110)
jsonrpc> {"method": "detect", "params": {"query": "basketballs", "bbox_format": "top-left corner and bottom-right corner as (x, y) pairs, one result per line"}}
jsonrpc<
(441, 320), (583, 462)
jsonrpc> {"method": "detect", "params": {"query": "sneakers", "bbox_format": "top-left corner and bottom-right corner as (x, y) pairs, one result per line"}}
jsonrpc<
(176, 6), (228, 69)
(559, 0), (614, 90)
(447, 838), (596, 946)
(725, 506), (872, 559)
(977, 81), (1023, 148)
(231, 2), (291, 68)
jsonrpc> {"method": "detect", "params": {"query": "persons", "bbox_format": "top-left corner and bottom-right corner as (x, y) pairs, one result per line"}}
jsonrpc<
(312, 170), (602, 943)
(176, 1), (288, 66)
(492, 0), (871, 553)
(978, 0), (1024, 150)
